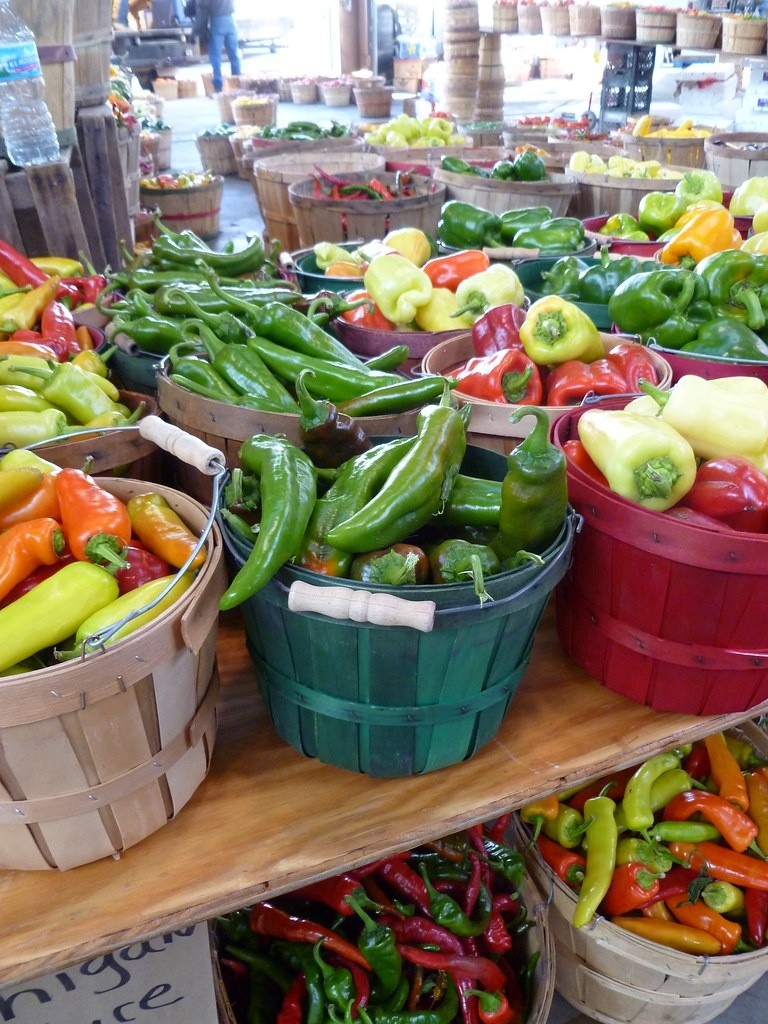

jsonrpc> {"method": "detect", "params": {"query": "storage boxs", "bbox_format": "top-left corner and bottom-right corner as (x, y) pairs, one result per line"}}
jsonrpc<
(535, 57), (573, 81)
(392, 59), (437, 95)
(597, 41), (656, 136)
(0, 917), (220, 1024)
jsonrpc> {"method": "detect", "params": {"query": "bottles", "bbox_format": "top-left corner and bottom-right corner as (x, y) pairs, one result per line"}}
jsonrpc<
(0, 1), (59, 167)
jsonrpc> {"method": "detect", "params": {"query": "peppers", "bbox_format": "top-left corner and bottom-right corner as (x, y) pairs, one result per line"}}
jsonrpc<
(0, 64), (768, 679)
(212, 731), (768, 1024)
(495, 0), (768, 20)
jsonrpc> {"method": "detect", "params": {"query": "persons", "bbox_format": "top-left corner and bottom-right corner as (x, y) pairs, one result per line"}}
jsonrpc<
(183, 0), (240, 92)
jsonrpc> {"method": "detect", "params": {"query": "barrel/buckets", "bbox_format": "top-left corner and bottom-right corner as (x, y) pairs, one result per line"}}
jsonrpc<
(507, 719), (768, 1024)
(206, 834), (556, 1024)
(1, 0), (767, 872)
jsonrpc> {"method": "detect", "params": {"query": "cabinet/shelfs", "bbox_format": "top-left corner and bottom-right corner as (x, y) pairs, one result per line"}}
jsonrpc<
(0, 618), (768, 996)
